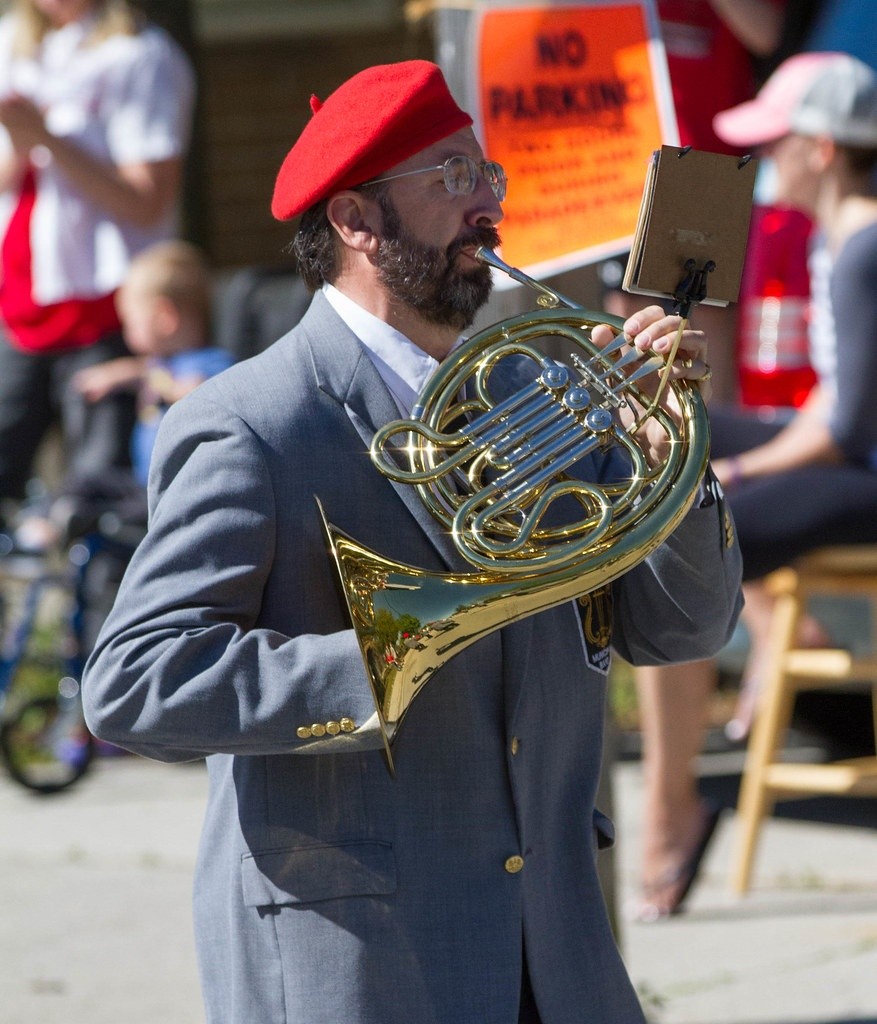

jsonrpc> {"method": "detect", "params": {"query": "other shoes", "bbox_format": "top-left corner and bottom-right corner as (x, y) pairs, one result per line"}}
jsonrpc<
(642, 791), (725, 915)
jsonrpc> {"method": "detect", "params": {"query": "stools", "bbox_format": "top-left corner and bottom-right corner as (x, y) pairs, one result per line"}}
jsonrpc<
(728, 545), (877, 895)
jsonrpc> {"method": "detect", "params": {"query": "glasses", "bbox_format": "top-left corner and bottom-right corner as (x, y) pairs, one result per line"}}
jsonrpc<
(349, 155), (509, 202)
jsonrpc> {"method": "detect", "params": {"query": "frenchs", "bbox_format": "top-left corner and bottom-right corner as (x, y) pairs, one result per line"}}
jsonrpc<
(310, 245), (714, 781)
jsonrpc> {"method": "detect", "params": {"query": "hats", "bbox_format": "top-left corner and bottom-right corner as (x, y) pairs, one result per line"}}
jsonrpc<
(271, 60), (475, 222)
(712, 51), (877, 150)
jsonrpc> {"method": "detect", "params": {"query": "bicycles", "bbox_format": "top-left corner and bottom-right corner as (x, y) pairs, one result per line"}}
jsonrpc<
(1, 377), (149, 796)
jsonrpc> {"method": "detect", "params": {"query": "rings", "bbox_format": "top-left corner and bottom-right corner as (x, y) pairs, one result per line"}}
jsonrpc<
(696, 363), (713, 383)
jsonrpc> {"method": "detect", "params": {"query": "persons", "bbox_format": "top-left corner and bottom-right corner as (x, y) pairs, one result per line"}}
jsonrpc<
(81, 59), (745, 1024)
(0, 0), (234, 490)
(642, 50), (877, 922)
